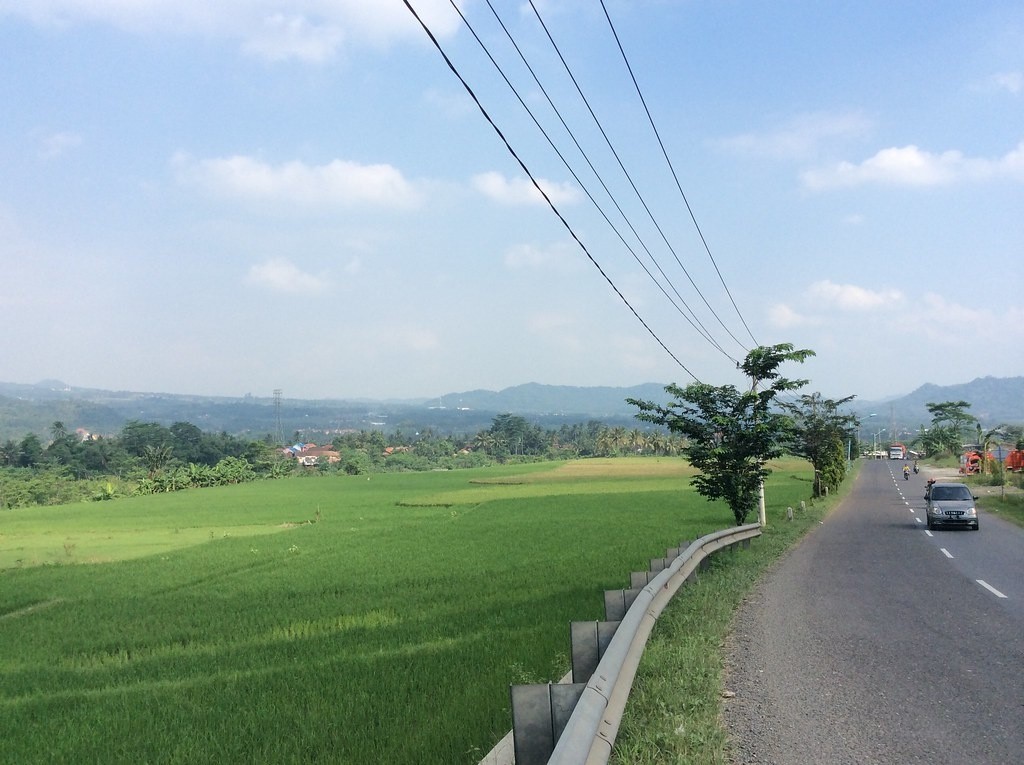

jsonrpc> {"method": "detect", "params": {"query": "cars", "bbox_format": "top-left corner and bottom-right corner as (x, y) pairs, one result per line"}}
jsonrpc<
(925, 482), (980, 530)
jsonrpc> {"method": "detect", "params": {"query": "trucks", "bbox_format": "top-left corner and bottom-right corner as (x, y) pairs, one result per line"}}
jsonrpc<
(889, 446), (906, 460)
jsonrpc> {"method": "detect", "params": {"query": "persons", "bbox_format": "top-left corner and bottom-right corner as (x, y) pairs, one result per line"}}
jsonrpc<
(913, 460), (920, 471)
(903, 464), (911, 475)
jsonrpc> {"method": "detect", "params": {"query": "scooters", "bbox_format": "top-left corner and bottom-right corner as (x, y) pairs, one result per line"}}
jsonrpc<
(914, 464), (919, 474)
(903, 469), (911, 481)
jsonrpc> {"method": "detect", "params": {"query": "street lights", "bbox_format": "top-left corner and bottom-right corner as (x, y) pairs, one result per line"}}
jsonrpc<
(846, 412), (877, 473)
(874, 428), (885, 460)
